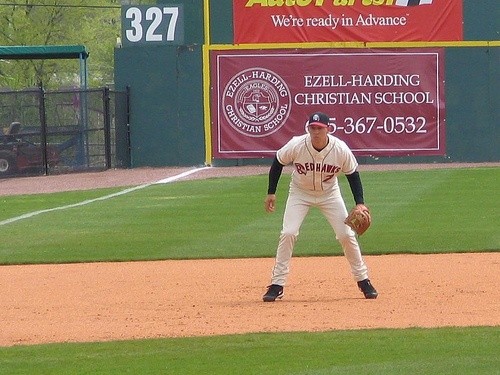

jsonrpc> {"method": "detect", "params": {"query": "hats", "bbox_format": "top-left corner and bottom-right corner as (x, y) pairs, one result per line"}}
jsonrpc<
(307, 112), (329, 127)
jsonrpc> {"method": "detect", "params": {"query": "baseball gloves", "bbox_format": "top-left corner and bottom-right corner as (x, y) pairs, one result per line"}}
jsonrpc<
(344, 208), (371, 236)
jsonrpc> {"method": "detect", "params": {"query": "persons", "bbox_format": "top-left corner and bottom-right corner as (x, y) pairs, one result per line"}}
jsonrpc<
(263, 112), (378, 302)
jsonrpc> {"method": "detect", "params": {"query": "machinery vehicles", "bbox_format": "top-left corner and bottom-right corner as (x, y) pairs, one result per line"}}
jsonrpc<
(0, 122), (58, 175)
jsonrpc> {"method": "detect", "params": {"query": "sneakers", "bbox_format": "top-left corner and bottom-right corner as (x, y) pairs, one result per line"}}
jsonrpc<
(263, 284), (284, 301)
(357, 279), (378, 298)
